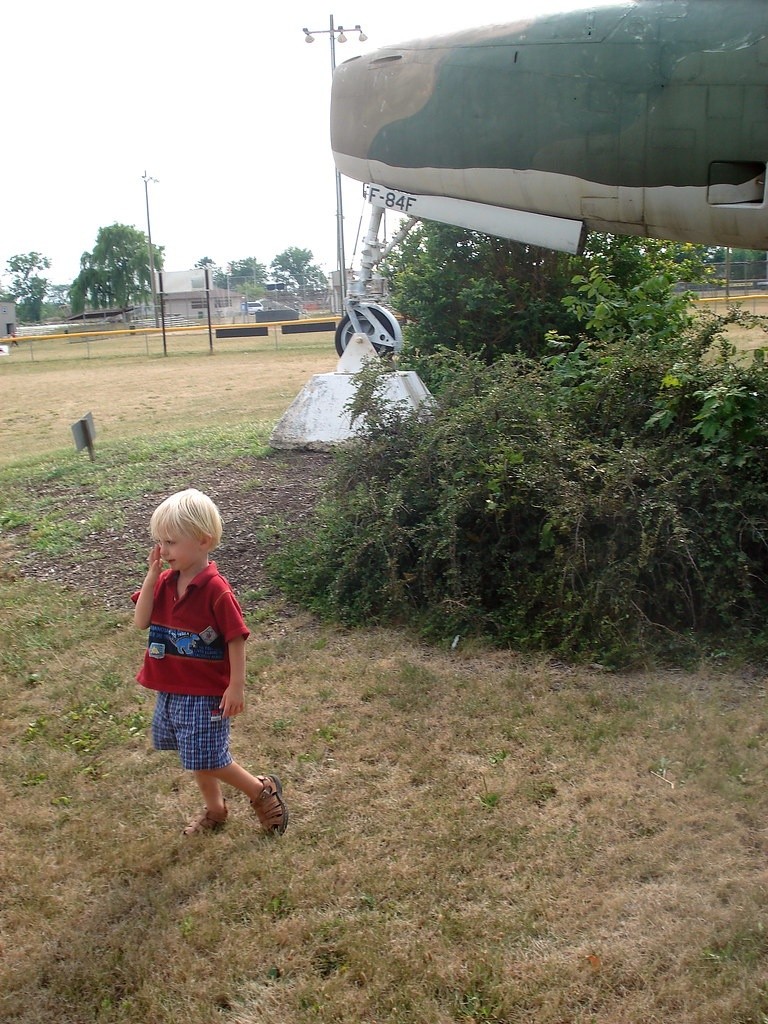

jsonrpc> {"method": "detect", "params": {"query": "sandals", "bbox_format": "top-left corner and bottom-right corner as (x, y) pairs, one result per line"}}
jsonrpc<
(249, 775), (288, 837)
(179, 799), (227, 842)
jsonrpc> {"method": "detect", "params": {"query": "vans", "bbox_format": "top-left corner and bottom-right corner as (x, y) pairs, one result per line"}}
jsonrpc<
(242, 301), (264, 314)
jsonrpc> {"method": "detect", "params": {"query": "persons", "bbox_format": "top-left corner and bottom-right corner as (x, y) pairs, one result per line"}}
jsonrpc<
(130, 487), (289, 838)
(10, 329), (19, 348)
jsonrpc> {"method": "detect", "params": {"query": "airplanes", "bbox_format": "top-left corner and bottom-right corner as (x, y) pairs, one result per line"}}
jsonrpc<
(333, 0), (767, 383)
